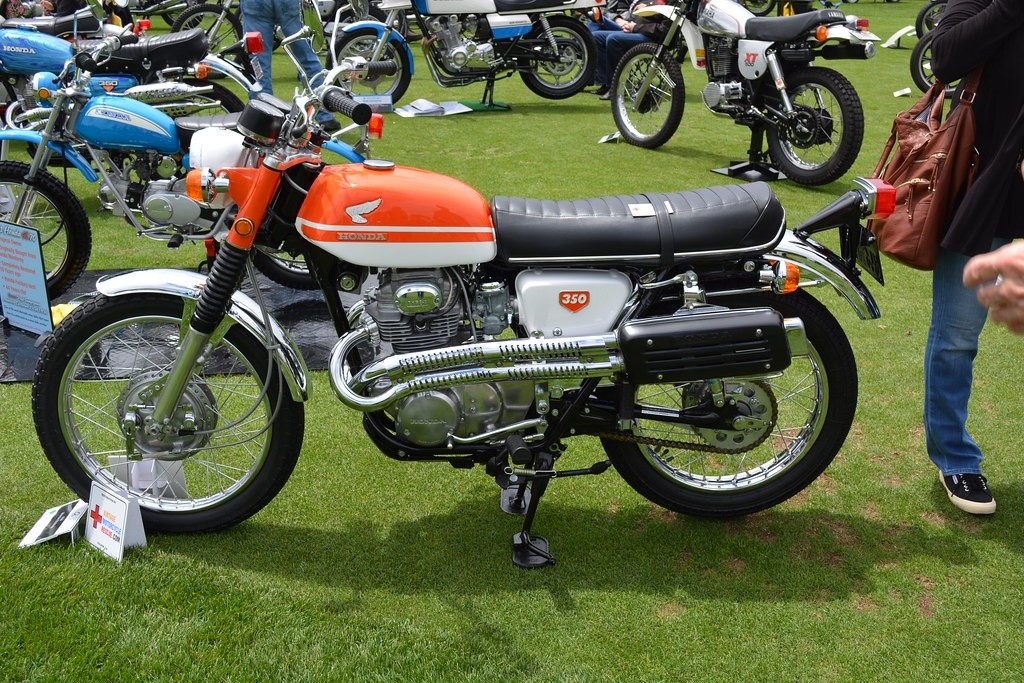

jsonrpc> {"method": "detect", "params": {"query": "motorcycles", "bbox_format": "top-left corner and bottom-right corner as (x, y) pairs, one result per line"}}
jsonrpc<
(1, 1), (961, 298)
(31, 0), (897, 567)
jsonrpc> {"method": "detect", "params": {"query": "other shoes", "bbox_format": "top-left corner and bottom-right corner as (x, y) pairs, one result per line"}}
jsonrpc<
(595, 84), (610, 94)
(600, 91), (612, 100)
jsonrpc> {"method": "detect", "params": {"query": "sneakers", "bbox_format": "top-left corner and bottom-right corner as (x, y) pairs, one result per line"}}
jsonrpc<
(939, 468), (997, 514)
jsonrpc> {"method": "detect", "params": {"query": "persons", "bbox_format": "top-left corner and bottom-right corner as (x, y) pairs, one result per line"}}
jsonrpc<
(0, 0), (86, 20)
(923, 0), (1024, 514)
(239, 0), (341, 132)
(103, 0), (134, 31)
(963, 239), (1024, 335)
(574, 0), (677, 100)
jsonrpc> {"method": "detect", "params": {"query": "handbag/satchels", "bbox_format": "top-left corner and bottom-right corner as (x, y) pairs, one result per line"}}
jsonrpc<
(868, 65), (980, 270)
